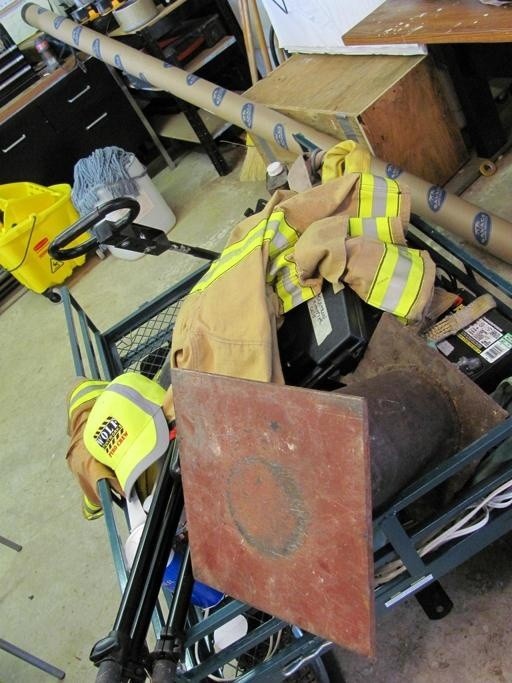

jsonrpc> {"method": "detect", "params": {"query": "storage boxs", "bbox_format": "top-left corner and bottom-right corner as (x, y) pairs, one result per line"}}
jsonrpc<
(240, 54), (470, 188)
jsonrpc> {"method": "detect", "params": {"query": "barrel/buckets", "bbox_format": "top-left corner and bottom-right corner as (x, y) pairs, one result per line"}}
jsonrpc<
(75, 152), (178, 262)
(1, 183), (92, 303)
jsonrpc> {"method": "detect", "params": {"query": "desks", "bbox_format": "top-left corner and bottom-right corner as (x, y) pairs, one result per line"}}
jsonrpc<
(341, 0), (512, 177)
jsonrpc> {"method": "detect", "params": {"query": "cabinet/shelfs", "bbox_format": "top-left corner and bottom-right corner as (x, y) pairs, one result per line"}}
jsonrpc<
(0, 56), (161, 191)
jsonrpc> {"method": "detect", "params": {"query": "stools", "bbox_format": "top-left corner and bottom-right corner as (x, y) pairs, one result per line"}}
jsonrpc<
(100, 1), (264, 177)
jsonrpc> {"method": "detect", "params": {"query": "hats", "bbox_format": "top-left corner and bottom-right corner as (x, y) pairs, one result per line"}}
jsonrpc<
(82, 371), (171, 501)
